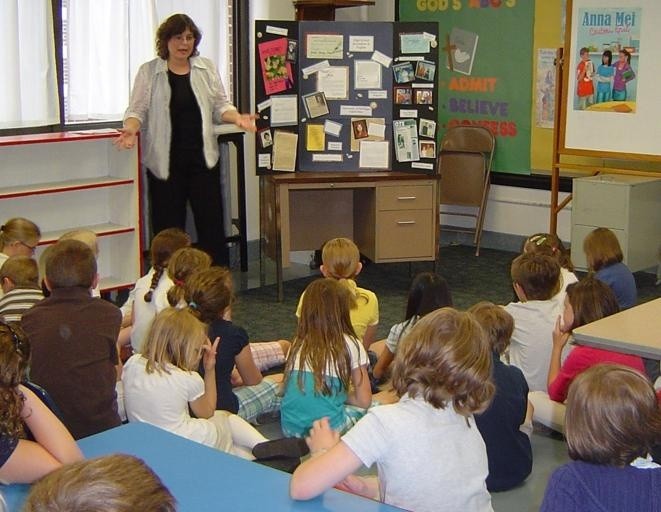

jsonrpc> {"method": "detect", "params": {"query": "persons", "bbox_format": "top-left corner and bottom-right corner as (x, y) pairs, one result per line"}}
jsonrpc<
(287, 43), (296, 61)
(593, 49), (616, 104)
(354, 122), (367, 138)
(279, 236), (532, 511)
(116, 228), (309, 474)
(23, 453), (176, 512)
(538, 363), (660, 510)
(1, 216), (123, 487)
(574, 47), (596, 110)
(611, 46), (637, 102)
(494, 226), (661, 432)
(111, 13), (262, 266)
(390, 59), (437, 160)
(262, 133), (272, 148)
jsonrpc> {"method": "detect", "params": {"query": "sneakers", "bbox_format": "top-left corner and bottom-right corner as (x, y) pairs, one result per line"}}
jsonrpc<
(252, 455), (300, 473)
(252, 436), (309, 459)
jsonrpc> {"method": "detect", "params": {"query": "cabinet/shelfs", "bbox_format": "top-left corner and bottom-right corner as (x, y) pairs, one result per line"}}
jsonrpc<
(0, 127), (144, 302)
(570, 174), (660, 280)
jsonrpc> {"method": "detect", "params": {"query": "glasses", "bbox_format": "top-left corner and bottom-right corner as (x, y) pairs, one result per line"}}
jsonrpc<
(20, 241), (36, 252)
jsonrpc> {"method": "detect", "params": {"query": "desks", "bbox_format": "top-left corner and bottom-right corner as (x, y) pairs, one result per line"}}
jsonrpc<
(260, 172), (442, 303)
(572, 295), (661, 361)
(0, 420), (408, 512)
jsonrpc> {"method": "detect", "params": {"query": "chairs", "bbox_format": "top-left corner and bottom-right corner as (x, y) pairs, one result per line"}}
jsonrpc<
(438, 125), (496, 257)
